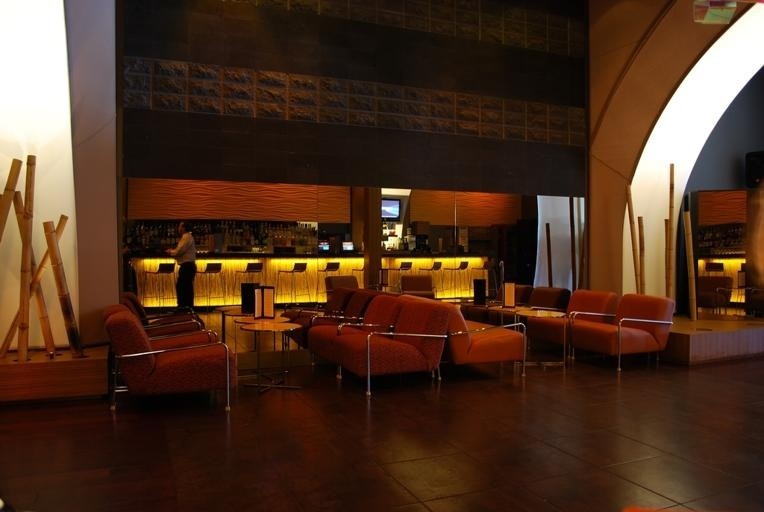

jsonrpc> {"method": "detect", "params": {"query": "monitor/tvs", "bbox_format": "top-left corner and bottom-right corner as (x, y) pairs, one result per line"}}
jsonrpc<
(381, 198), (401, 221)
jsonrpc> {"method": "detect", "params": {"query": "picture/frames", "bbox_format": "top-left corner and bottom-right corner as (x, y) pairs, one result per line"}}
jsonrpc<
(738, 271), (746, 288)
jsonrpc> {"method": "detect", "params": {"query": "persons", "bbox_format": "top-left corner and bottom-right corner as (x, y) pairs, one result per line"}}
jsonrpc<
(163, 220), (197, 315)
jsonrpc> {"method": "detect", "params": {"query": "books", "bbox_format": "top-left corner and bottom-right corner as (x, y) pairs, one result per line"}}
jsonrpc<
(501, 280), (516, 308)
(474, 278), (486, 305)
(241, 282), (260, 314)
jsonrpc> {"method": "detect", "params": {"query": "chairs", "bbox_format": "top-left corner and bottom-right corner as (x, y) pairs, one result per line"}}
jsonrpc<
(695, 276), (733, 318)
(102, 260), (237, 413)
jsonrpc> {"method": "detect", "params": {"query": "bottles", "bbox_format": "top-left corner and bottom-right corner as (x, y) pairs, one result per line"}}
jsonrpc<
(127, 220), (319, 255)
